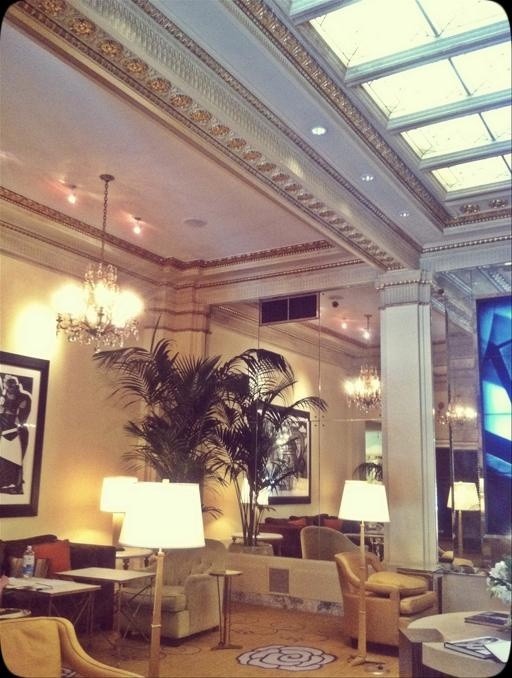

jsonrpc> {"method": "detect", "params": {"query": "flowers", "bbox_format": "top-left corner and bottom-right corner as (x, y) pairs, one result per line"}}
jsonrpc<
(478, 556), (512, 606)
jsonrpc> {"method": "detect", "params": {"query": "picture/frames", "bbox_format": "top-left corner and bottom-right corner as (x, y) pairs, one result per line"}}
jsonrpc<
(0, 350), (50, 518)
(254, 400), (311, 504)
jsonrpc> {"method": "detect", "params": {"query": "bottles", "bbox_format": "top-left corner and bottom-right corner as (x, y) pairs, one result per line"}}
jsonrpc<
(23, 545), (34, 579)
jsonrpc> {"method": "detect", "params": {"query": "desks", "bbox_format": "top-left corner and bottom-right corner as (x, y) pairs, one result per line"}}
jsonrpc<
(56, 566), (156, 648)
(397, 611), (512, 678)
(113, 547), (152, 570)
(230, 532), (283, 556)
(2, 574), (101, 652)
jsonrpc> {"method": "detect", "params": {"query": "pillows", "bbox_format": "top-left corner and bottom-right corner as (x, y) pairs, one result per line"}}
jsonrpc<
(313, 513), (328, 525)
(290, 514), (313, 525)
(367, 571), (428, 596)
(265, 517), (286, 525)
(324, 518), (343, 532)
(287, 519), (305, 528)
(29, 540), (73, 581)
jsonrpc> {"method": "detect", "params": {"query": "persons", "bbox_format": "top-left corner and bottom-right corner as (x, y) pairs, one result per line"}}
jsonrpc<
(0, 376), (32, 495)
(0, 574), (9, 596)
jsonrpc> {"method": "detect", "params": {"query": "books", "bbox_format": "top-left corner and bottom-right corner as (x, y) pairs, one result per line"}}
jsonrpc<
(443, 636), (512, 665)
(12, 582), (53, 591)
(464, 610), (510, 628)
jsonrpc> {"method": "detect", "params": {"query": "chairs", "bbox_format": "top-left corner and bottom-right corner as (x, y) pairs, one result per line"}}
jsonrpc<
(0, 616), (142, 678)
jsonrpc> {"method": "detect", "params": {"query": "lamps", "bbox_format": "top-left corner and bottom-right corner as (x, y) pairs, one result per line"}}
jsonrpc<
(99, 475), (138, 548)
(240, 477), (269, 539)
(46, 173), (141, 354)
(446, 481), (481, 557)
(341, 314), (382, 416)
(117, 479), (205, 677)
(439, 363), (478, 429)
(337, 479), (391, 667)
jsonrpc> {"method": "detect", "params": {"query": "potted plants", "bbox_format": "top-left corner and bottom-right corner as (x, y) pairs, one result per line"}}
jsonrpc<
(210, 360), (328, 554)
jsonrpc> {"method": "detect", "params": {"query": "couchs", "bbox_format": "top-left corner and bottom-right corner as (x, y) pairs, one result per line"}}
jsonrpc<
(259, 516), (360, 557)
(335, 547), (436, 651)
(118, 537), (226, 645)
(300, 526), (359, 560)
(2, 541), (117, 631)
